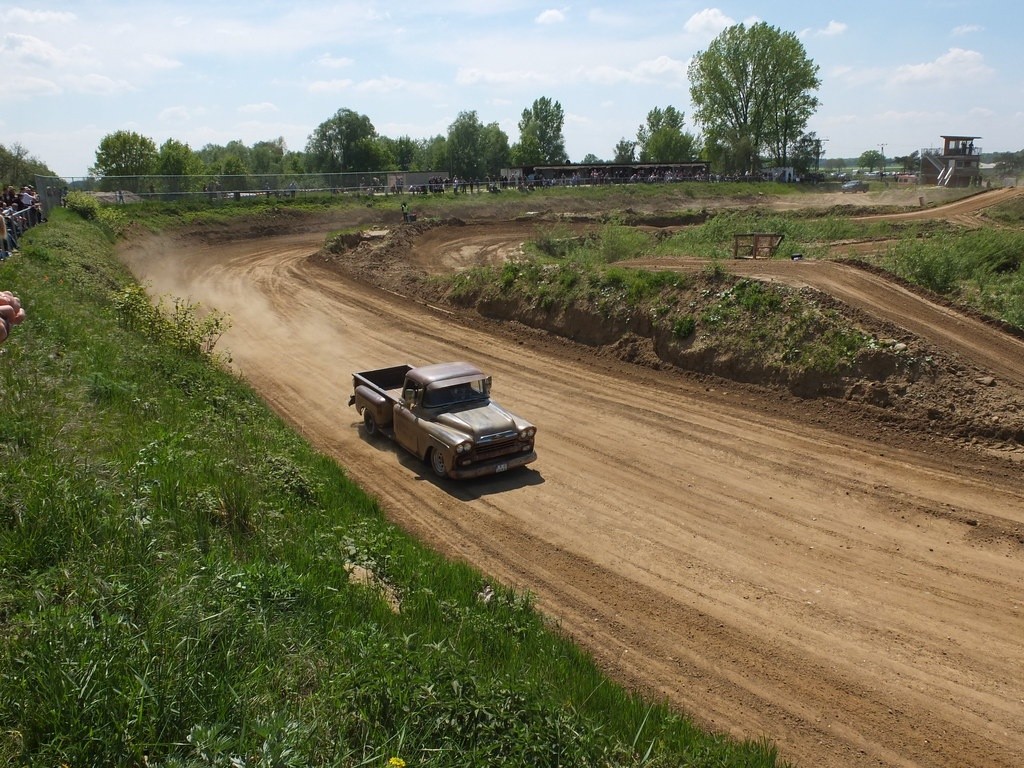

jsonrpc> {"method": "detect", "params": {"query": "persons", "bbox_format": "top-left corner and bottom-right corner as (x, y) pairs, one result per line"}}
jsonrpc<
(263, 181), (270, 190)
(469, 169), (824, 190)
(0, 291), (26, 345)
(290, 182), (296, 198)
(203, 184), (207, 192)
(118, 190), (123, 204)
(391, 175), (466, 194)
(402, 203), (409, 222)
(962, 142), (966, 155)
(0, 185), (68, 260)
(968, 142), (973, 155)
(360, 177), (365, 190)
(150, 183), (155, 194)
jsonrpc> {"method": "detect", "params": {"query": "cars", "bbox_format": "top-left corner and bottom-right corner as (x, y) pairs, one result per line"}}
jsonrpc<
(831, 170), (910, 178)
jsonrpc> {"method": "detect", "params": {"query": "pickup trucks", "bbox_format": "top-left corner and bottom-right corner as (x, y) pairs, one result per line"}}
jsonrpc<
(347, 361), (538, 480)
(841, 180), (869, 193)
